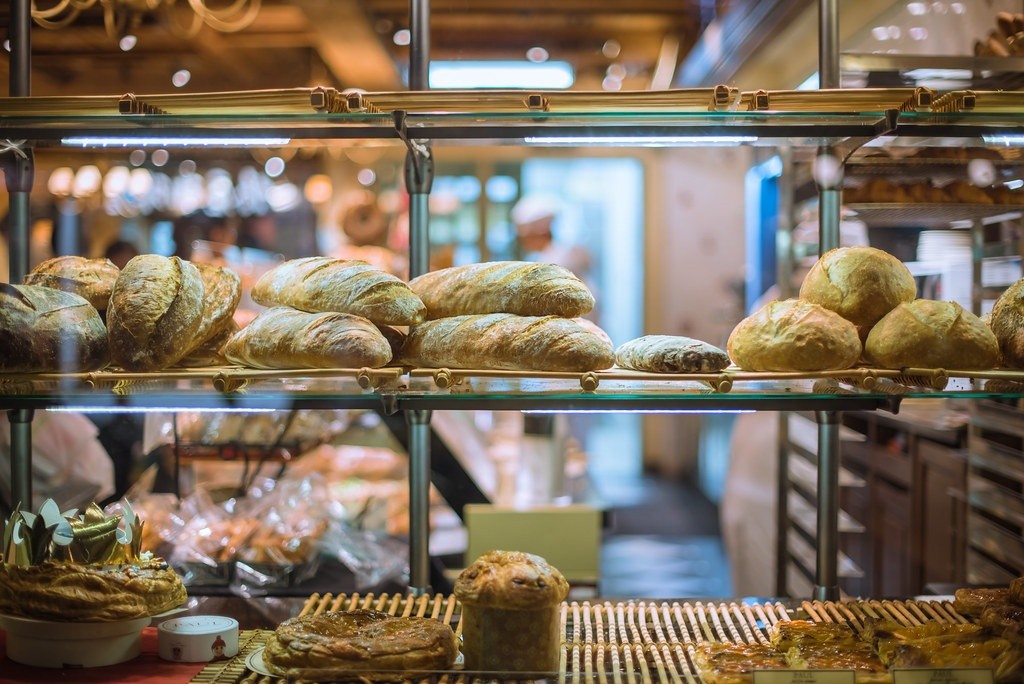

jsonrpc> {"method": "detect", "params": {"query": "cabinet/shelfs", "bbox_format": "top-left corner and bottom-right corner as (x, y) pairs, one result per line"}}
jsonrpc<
(0, 80), (1022, 413)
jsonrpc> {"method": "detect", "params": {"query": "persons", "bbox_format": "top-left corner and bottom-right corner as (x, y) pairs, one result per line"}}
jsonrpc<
(0, 408), (116, 520)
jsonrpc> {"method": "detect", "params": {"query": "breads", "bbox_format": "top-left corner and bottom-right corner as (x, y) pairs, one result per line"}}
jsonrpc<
(693, 575), (1024, 684)
(453, 550), (570, 679)
(0, 248), (244, 368)
(263, 609), (459, 682)
(224, 255), (615, 371)
(726, 244), (1024, 373)
(0, 554), (189, 622)
(615, 334), (731, 372)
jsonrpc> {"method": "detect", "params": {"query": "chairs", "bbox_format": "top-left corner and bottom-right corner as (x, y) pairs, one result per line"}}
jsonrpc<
(465, 507), (604, 599)
